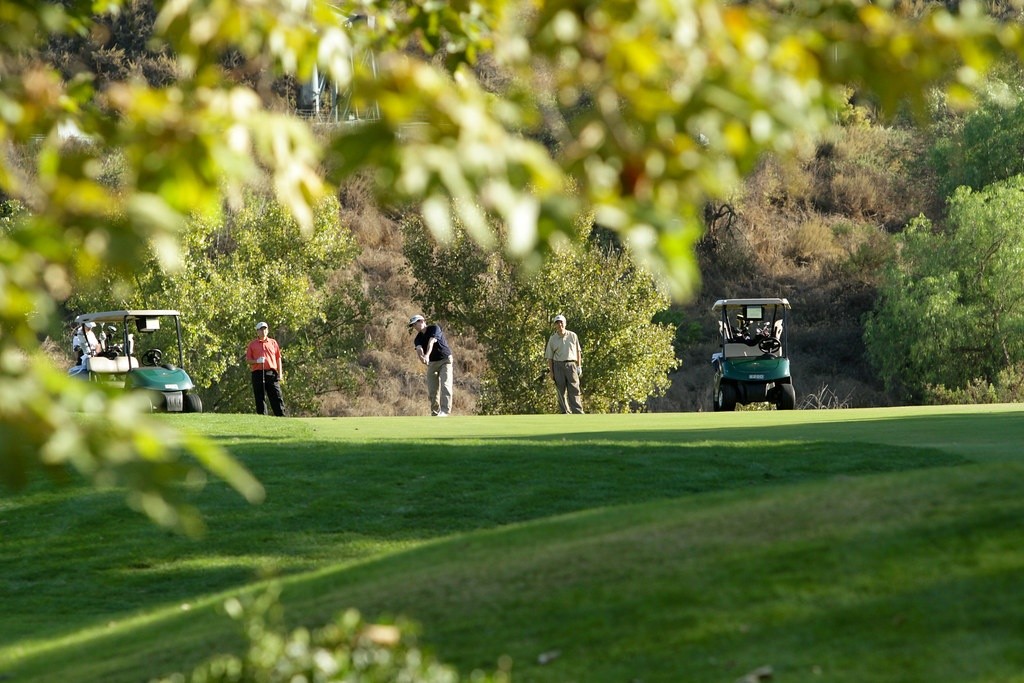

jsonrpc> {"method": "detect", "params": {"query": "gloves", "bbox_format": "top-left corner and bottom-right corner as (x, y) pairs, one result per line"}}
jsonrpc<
(577, 367), (582, 377)
(257, 357), (265, 363)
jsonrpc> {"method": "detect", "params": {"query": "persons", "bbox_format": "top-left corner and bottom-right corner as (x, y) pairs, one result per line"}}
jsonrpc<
(545, 315), (585, 414)
(73, 321), (100, 366)
(409, 315), (453, 416)
(246, 322), (286, 417)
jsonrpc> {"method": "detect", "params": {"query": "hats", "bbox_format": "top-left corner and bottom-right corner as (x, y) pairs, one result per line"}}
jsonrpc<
(409, 315), (424, 327)
(256, 322), (268, 330)
(84, 322), (96, 328)
(554, 315), (566, 323)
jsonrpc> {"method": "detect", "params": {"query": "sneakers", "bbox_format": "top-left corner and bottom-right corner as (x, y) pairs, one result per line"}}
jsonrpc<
(430, 410), (449, 417)
